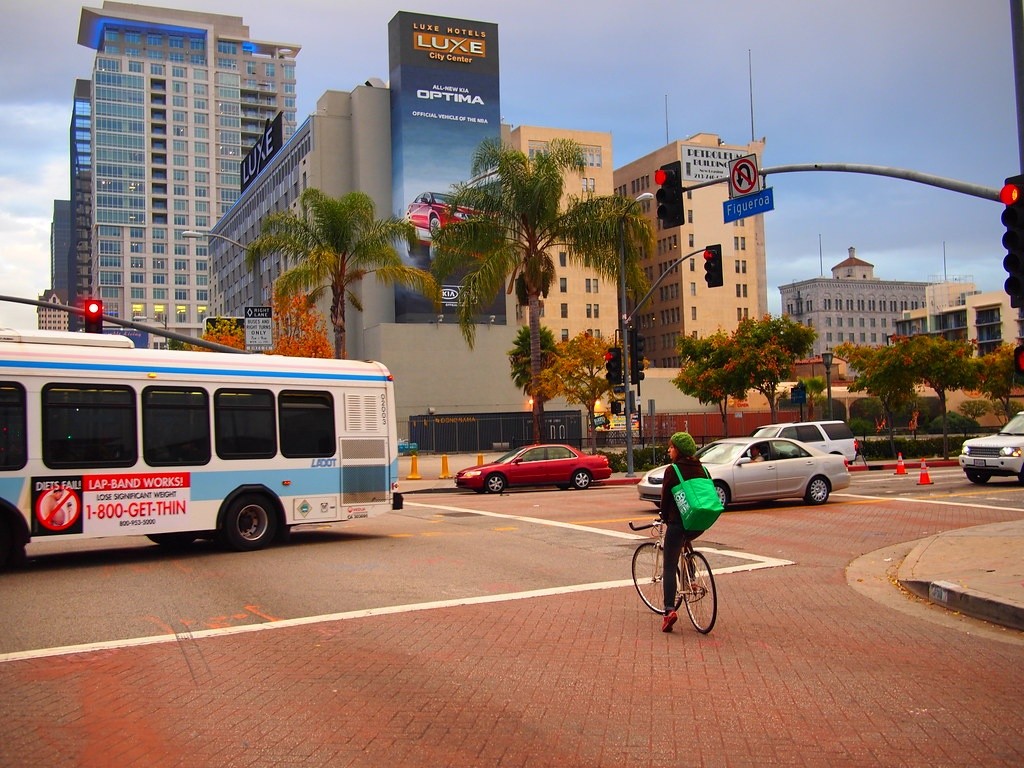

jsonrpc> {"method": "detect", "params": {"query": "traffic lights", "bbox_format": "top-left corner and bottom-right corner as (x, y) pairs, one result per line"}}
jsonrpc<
(1013, 344), (1024, 383)
(629, 326), (646, 385)
(702, 244), (725, 289)
(655, 160), (684, 229)
(85, 299), (104, 335)
(999, 172), (1024, 310)
(607, 347), (623, 385)
(611, 401), (621, 414)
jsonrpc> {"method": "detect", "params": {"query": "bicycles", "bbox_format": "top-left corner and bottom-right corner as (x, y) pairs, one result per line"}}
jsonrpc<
(629, 512), (718, 634)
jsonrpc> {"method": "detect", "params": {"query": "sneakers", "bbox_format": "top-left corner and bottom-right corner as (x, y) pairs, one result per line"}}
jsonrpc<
(688, 583), (698, 591)
(661, 611), (678, 632)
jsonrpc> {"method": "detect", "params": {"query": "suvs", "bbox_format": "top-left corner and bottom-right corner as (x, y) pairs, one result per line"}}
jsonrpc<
(958, 412), (1024, 488)
(742, 420), (859, 466)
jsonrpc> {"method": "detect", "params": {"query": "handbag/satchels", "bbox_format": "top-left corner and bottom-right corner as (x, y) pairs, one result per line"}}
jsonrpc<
(671, 462), (724, 531)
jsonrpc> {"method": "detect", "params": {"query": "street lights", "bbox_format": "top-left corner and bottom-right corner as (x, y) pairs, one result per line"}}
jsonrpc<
(822, 351), (834, 420)
(182, 231), (262, 306)
(614, 191), (655, 479)
(132, 314), (168, 350)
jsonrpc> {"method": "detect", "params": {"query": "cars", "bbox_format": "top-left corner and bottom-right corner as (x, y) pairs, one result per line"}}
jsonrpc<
(452, 445), (615, 497)
(634, 437), (853, 508)
(407, 193), (488, 240)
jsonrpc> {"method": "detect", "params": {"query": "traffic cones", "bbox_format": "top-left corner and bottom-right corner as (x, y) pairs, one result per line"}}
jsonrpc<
(893, 452), (908, 476)
(916, 457), (933, 486)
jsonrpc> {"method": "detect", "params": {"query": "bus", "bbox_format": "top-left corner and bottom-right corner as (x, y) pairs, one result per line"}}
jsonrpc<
(0, 330), (404, 559)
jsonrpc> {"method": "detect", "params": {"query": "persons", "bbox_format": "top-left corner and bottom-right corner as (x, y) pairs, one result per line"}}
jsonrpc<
(749, 445), (764, 463)
(659, 432), (712, 633)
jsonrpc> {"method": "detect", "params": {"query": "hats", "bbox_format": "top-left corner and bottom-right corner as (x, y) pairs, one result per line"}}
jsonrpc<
(671, 432), (697, 456)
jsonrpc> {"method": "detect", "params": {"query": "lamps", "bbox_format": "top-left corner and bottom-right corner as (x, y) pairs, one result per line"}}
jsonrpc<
(437, 314), (444, 323)
(489, 314), (496, 323)
(428, 407), (436, 414)
(364, 77), (386, 89)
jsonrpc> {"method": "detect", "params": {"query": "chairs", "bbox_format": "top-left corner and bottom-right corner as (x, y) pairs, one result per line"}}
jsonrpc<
(791, 449), (799, 457)
(780, 450), (790, 459)
(760, 446), (769, 460)
